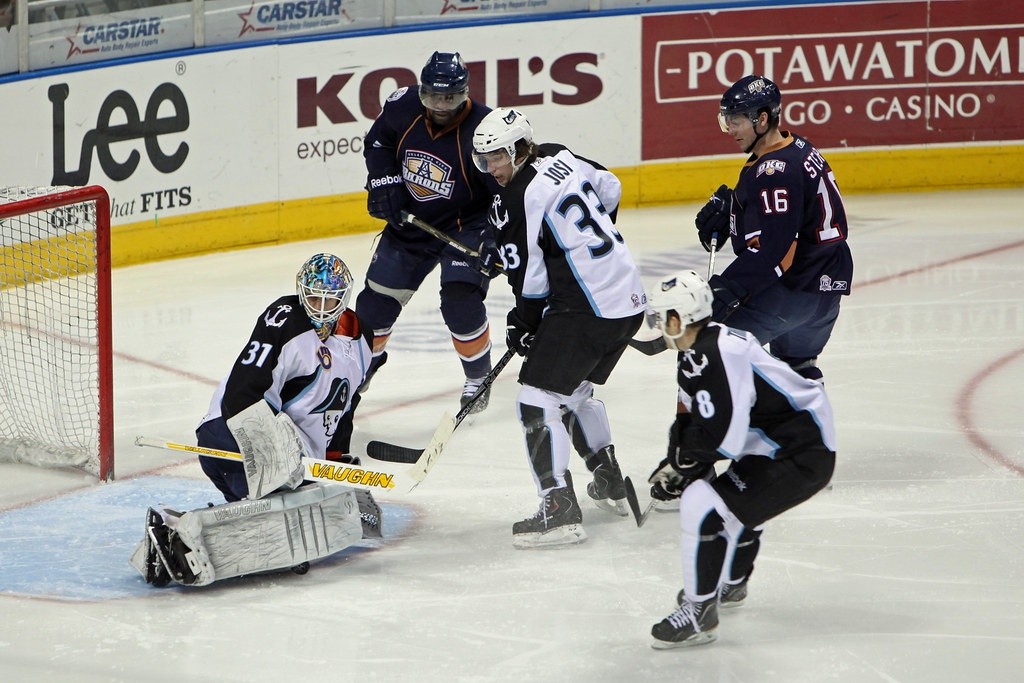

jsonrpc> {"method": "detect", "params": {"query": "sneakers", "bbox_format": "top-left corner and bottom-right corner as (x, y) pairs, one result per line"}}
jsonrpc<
(510, 471), (588, 547)
(460, 377), (491, 425)
(651, 582), (720, 650)
(651, 481), (681, 511)
(676, 565), (754, 611)
(585, 445), (628, 517)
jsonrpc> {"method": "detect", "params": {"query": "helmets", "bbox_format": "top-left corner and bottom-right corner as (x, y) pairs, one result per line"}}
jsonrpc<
(420, 51), (469, 96)
(297, 254), (352, 341)
(646, 271), (713, 329)
(718, 76), (781, 123)
(473, 107), (534, 157)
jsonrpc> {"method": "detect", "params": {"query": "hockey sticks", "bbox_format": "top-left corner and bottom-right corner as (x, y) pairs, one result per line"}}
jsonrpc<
(624, 473), (657, 530)
(398, 210), (669, 358)
(364, 346), (517, 465)
(134, 409), (459, 499)
(708, 231), (718, 283)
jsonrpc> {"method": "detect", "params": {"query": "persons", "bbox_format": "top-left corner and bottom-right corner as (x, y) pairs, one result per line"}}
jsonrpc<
(644, 268), (837, 648)
(471, 107), (648, 548)
(129, 252), (385, 586)
(654, 75), (853, 512)
(355, 52), (501, 426)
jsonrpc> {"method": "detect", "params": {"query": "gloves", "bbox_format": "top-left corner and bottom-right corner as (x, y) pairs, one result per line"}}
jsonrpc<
(505, 310), (538, 358)
(694, 185), (736, 253)
(649, 443), (707, 499)
(466, 227), (503, 278)
(366, 174), (406, 232)
(707, 276), (742, 321)
